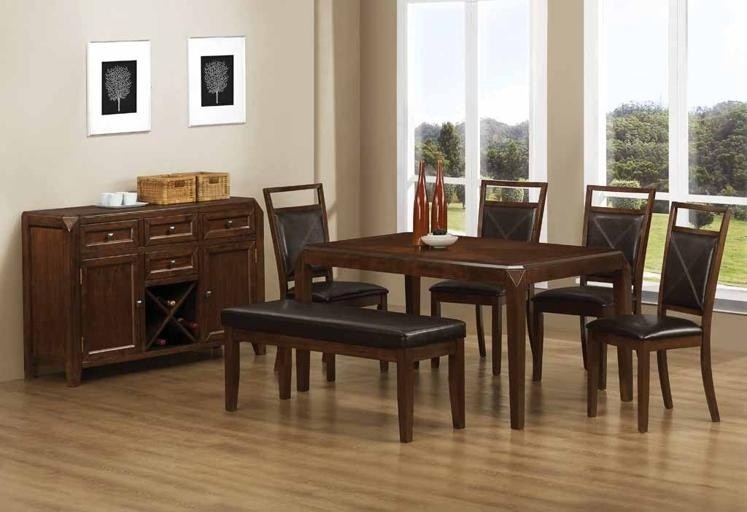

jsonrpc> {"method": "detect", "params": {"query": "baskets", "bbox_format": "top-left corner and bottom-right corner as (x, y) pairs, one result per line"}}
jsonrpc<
(196, 172), (231, 202)
(136, 172), (198, 205)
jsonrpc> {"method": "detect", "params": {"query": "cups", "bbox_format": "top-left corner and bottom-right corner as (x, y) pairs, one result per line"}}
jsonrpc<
(101, 192), (137, 205)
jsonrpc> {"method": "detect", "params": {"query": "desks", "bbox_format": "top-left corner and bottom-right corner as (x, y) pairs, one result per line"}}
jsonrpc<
(294, 232), (633, 429)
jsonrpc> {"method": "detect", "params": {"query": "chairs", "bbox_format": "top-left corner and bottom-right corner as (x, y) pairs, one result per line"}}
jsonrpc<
(262, 182), (388, 382)
(532, 185), (657, 391)
(583, 202), (735, 432)
(431, 179), (550, 376)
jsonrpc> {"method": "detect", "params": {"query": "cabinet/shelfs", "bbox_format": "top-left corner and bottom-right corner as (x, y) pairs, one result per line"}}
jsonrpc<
(20, 198), (267, 387)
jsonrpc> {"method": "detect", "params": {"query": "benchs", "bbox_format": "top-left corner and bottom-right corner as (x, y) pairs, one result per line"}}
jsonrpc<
(221, 300), (467, 444)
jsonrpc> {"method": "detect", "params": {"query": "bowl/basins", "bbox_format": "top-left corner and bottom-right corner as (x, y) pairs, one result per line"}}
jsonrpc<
(421, 235), (459, 248)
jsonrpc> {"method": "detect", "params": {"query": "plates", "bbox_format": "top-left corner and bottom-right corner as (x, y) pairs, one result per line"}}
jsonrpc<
(93, 201), (149, 208)
